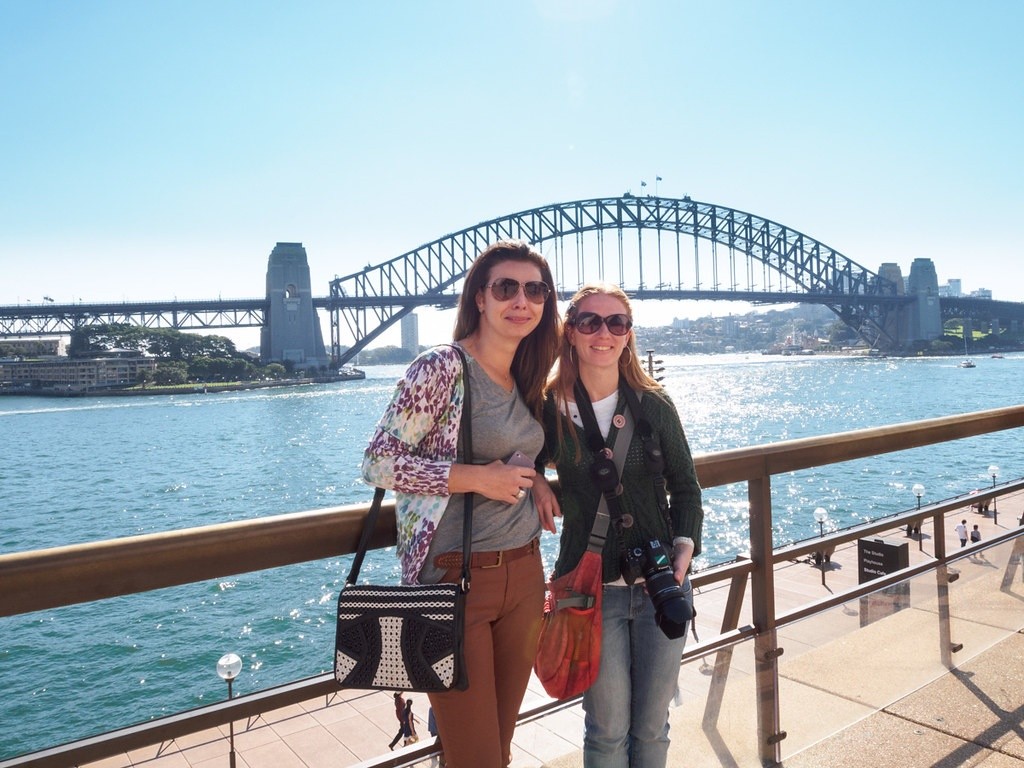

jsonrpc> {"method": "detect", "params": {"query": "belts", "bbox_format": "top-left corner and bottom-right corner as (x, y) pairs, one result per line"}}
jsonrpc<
(434, 537), (540, 569)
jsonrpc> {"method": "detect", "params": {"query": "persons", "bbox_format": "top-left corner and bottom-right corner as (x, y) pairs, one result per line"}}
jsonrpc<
(530, 279), (705, 767)
(359, 241), (562, 768)
(955, 519), (969, 548)
(969, 524), (985, 560)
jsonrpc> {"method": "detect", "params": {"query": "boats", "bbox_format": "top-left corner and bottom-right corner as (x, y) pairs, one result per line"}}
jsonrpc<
(991, 354), (1003, 359)
(761, 345), (816, 356)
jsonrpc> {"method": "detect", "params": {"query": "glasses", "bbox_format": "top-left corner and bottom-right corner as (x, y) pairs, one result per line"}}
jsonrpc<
(483, 277), (552, 305)
(571, 311), (633, 336)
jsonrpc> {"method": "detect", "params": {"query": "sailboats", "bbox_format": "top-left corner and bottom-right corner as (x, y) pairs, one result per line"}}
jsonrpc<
(961, 338), (975, 367)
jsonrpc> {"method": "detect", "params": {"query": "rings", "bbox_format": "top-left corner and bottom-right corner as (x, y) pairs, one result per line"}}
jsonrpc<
(515, 490), (526, 499)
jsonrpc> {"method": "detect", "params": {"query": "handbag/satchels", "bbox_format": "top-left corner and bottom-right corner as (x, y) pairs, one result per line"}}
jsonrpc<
(971, 536), (979, 543)
(406, 735), (419, 745)
(332, 583), (467, 693)
(534, 549), (603, 701)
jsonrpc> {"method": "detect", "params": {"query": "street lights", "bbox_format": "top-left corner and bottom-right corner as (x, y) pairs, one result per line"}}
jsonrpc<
(988, 465), (999, 524)
(216, 653), (243, 768)
(913, 484), (924, 551)
(814, 507), (830, 585)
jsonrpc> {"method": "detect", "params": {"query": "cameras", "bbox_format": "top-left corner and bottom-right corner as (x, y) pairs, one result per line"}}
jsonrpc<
(618, 539), (696, 640)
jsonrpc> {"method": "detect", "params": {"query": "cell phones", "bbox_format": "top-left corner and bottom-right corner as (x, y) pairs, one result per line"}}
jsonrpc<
(499, 451), (536, 506)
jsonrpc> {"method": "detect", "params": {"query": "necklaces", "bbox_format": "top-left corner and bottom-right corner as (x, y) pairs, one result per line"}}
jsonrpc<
(466, 334), (513, 382)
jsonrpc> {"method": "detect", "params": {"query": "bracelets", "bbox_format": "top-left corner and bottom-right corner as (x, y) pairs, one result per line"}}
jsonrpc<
(671, 535), (695, 551)
